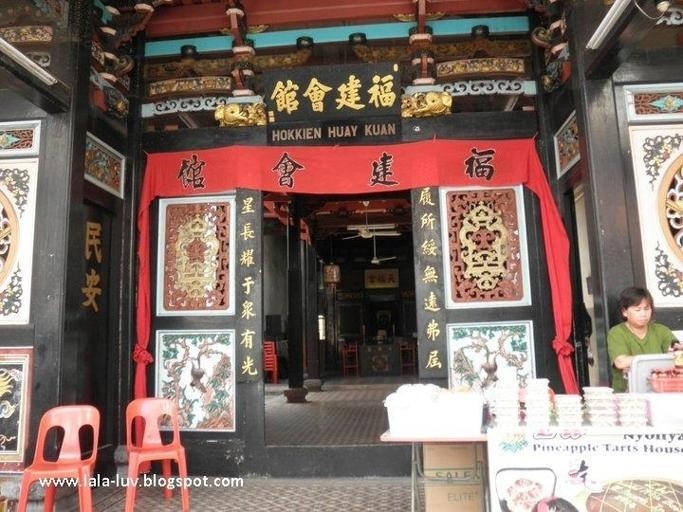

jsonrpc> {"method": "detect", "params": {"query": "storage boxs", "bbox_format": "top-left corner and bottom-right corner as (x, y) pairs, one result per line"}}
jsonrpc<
(423, 442), (485, 511)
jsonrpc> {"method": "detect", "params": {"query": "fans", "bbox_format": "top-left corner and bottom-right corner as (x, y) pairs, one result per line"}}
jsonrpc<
(341, 201), (402, 240)
(353, 230), (397, 265)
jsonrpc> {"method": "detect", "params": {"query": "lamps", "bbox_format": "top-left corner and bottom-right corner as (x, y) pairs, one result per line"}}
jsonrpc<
(347, 224), (398, 230)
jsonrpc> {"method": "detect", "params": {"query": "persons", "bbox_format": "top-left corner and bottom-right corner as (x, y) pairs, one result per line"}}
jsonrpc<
(604, 287), (683, 392)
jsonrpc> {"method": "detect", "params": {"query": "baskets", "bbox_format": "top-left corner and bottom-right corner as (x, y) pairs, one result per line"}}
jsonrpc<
(647, 368), (683, 392)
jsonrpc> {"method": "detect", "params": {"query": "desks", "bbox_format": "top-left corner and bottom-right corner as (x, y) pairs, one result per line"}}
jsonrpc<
(487, 427), (683, 511)
(380, 428), (488, 512)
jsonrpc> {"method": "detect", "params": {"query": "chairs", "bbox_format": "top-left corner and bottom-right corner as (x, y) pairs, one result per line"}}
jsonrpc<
(125, 397), (189, 512)
(264, 341), (277, 384)
(17, 404), (100, 511)
